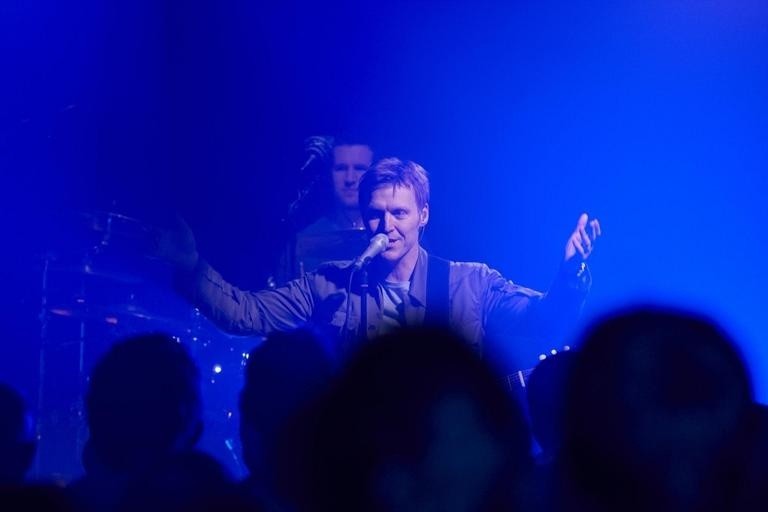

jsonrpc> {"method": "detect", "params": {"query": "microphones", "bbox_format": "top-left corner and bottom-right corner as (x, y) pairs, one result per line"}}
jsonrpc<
(352, 233), (389, 270)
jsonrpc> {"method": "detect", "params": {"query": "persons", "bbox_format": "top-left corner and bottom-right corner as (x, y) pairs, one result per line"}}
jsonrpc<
(292, 131), (383, 272)
(158, 159), (602, 367)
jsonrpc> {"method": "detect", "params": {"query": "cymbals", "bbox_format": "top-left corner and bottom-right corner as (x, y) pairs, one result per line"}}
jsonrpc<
(97, 306), (170, 324)
(90, 210), (138, 220)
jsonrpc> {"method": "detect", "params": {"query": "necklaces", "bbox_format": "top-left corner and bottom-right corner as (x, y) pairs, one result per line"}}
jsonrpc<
(341, 210), (361, 230)
